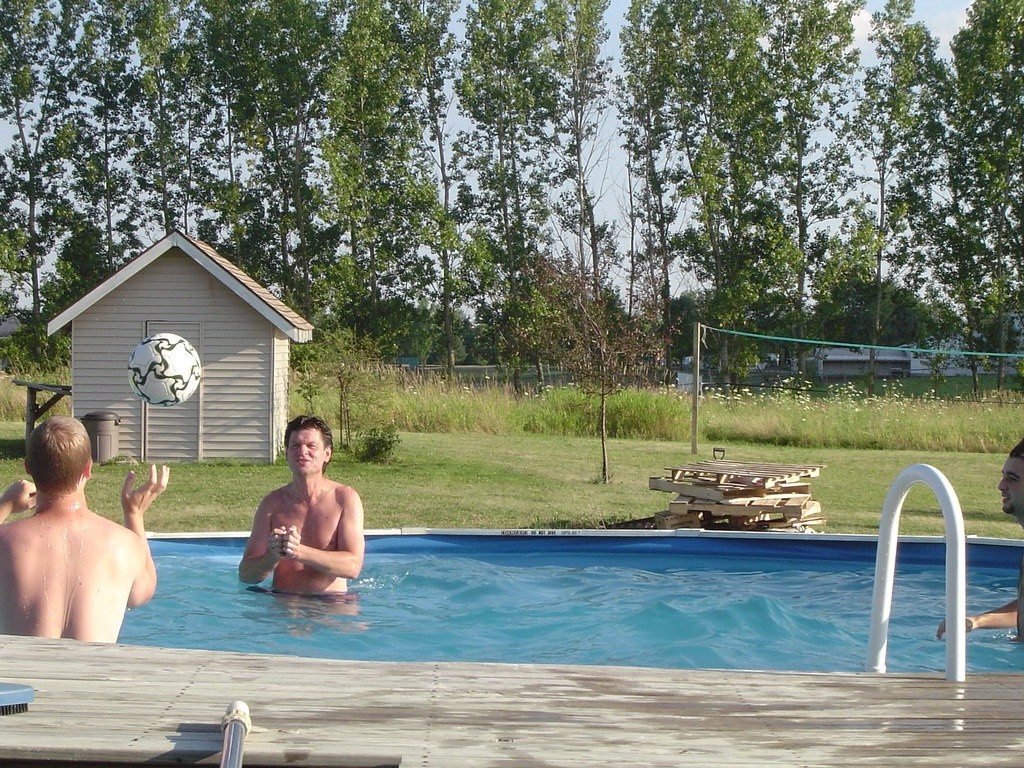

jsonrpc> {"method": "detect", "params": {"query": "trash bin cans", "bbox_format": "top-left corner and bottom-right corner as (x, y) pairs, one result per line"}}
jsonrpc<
(81, 410), (122, 468)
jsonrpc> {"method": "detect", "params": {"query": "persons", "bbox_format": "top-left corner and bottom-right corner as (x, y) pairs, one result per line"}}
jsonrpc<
(937, 440), (1024, 640)
(238, 414), (366, 597)
(0, 415), (170, 644)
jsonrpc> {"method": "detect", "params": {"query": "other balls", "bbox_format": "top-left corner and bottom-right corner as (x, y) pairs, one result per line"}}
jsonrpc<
(124, 331), (206, 410)
